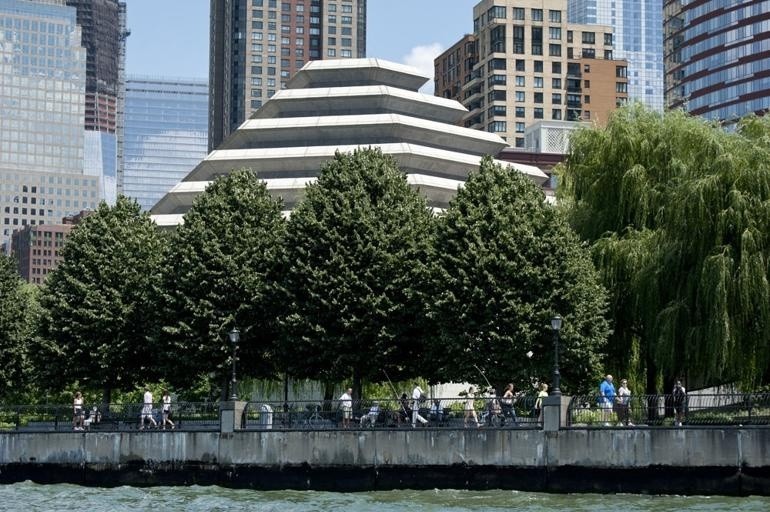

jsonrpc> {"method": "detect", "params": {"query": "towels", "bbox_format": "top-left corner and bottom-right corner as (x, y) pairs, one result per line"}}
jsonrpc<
(260, 404), (273, 429)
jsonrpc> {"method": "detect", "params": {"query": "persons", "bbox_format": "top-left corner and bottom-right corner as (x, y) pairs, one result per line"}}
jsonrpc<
(73, 392), (85, 431)
(161, 391), (175, 431)
(339, 388), (353, 430)
(672, 381), (685, 426)
(140, 387), (157, 431)
(360, 401), (381, 430)
(597, 375), (621, 426)
(616, 379), (636, 427)
(83, 406), (102, 431)
(399, 382), (549, 427)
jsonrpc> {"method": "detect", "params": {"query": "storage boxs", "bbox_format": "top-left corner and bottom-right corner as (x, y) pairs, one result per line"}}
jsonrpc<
(123, 417), (158, 429)
(90, 417), (119, 431)
(419, 407), (451, 427)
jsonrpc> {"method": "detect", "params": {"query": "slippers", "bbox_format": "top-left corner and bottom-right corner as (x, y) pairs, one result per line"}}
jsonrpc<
(416, 389), (426, 403)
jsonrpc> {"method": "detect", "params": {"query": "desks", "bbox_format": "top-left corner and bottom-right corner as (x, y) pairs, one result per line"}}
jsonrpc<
(228, 327), (241, 402)
(551, 312), (564, 396)
